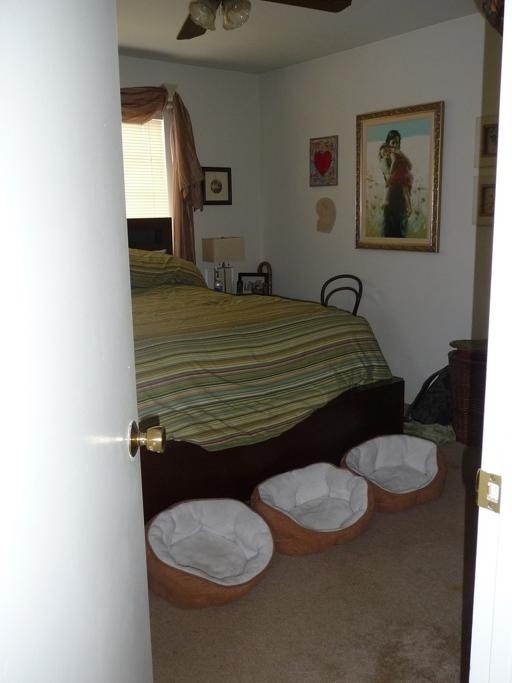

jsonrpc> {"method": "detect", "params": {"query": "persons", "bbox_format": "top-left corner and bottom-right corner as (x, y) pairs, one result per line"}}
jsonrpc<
(366, 129), (414, 237)
(379, 143), (415, 217)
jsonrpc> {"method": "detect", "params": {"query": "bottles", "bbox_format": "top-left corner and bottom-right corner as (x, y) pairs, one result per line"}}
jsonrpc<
(214, 277), (224, 292)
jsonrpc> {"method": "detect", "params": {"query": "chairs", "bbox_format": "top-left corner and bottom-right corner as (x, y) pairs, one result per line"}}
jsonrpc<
(321, 274), (363, 317)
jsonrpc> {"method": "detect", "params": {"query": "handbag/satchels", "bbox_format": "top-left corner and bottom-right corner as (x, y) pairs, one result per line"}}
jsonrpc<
(404, 365), (452, 426)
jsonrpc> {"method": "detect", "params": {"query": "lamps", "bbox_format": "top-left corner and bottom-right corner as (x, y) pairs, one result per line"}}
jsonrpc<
(187, 0), (251, 31)
(202, 236), (245, 295)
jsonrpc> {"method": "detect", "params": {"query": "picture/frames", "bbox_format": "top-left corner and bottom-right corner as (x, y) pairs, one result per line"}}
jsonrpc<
(473, 114), (498, 168)
(200, 167), (232, 205)
(355, 101), (444, 253)
(473, 176), (495, 226)
(237, 272), (272, 296)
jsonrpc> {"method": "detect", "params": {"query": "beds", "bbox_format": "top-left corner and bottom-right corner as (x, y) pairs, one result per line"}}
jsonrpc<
(127, 218), (405, 523)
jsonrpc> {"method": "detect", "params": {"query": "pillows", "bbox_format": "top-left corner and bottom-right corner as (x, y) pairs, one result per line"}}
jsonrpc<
(129, 248), (209, 288)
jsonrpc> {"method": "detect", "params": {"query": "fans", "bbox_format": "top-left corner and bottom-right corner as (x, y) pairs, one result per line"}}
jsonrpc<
(176, 0), (352, 40)
